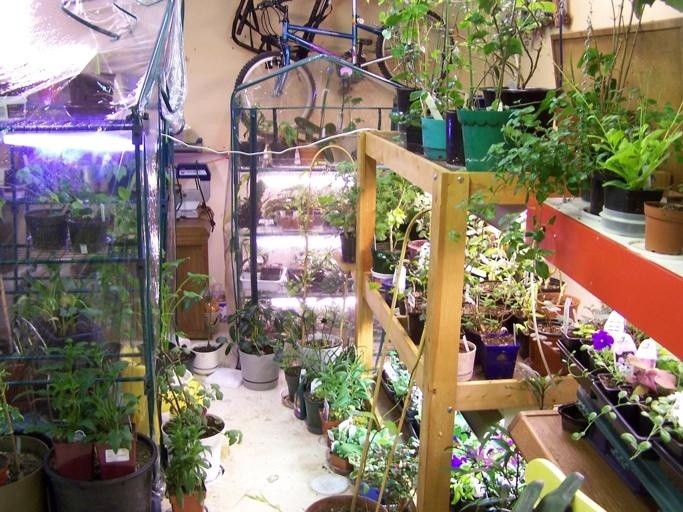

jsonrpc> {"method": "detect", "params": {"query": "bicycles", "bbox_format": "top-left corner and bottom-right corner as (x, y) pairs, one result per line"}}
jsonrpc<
(234, 0), (455, 142)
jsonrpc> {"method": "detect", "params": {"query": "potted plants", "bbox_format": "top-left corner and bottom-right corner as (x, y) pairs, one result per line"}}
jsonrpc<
(0, 147), (242, 512)
(224, 92), (368, 293)
(377, 0), (682, 511)
(218, 298), (420, 511)
